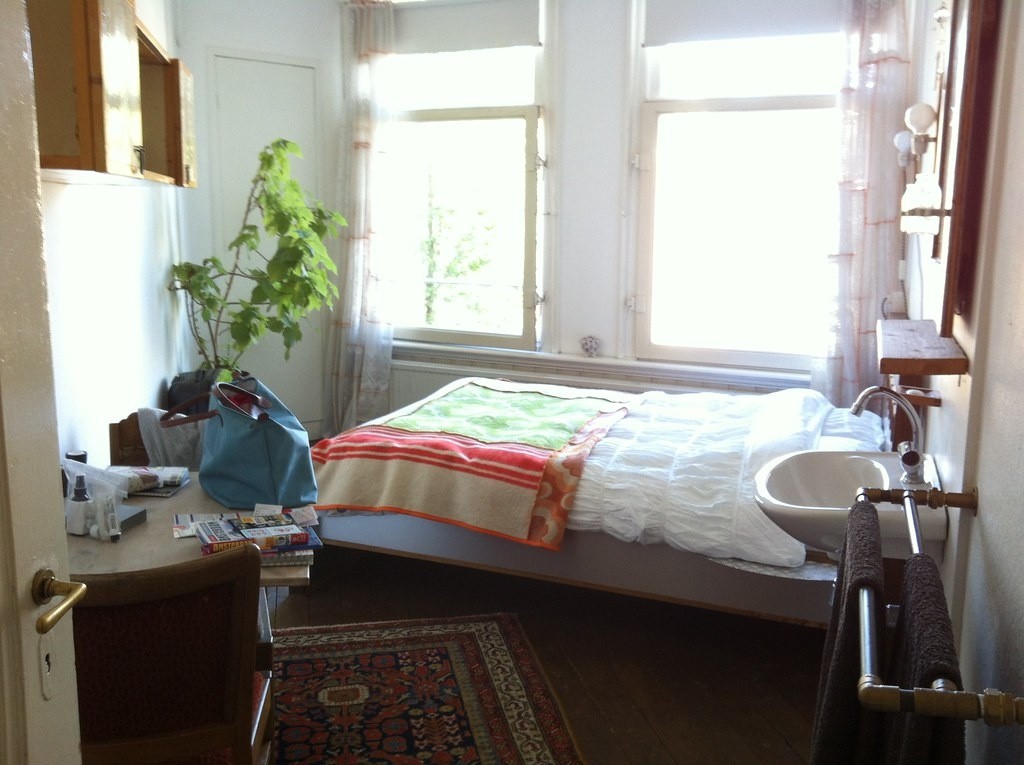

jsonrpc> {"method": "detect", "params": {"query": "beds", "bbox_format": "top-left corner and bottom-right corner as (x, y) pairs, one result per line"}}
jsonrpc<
(314, 378), (893, 632)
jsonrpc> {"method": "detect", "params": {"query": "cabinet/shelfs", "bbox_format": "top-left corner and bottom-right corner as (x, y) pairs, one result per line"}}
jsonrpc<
(27, 0), (202, 191)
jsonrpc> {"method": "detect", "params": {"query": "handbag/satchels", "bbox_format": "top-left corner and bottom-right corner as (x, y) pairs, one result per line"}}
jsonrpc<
(158, 373), (319, 511)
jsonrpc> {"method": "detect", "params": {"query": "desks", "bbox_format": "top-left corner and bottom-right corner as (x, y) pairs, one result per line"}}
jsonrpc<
(68, 472), (311, 668)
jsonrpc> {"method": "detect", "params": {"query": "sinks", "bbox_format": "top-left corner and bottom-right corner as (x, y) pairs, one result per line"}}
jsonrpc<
(753, 450), (899, 558)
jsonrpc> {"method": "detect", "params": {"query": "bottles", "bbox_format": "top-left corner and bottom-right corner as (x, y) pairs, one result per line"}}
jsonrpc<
(900, 170), (942, 235)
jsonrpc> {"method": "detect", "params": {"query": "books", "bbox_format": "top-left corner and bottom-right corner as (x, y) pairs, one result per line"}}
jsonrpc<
(193, 514), (322, 569)
(107, 465), (189, 500)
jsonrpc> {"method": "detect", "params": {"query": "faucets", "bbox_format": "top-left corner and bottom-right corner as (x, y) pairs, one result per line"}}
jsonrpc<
(850, 385), (927, 484)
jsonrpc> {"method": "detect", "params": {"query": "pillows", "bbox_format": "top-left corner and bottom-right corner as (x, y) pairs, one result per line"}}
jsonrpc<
(818, 407), (885, 452)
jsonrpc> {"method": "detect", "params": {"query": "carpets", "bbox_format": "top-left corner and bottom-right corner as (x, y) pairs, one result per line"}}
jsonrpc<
(272, 610), (590, 765)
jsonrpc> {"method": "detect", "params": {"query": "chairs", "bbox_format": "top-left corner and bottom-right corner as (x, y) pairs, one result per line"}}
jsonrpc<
(110, 409), (203, 472)
(70, 544), (274, 765)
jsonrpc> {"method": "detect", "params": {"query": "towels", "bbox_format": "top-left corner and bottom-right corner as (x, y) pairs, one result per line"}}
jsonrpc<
(889, 552), (968, 764)
(807, 502), (886, 765)
